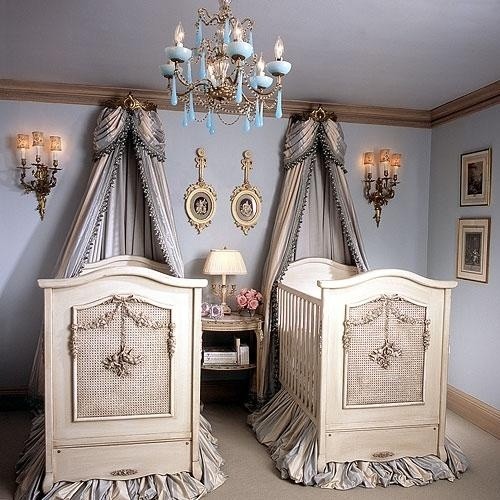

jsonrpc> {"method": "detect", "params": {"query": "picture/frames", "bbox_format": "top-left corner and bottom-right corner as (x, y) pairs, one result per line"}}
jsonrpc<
(183, 146), (218, 234)
(230, 149), (263, 236)
(456, 217), (491, 283)
(459, 145), (493, 207)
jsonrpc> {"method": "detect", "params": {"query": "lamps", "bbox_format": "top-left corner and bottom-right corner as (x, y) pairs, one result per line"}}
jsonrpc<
(202, 248), (249, 313)
(157, 0), (290, 135)
(16, 130), (63, 221)
(362, 148), (403, 228)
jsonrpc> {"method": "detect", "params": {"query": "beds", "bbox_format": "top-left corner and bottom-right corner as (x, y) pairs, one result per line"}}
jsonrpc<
(274, 256), (457, 479)
(37, 254), (208, 500)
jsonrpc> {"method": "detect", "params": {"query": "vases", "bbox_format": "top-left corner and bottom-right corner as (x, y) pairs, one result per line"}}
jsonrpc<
(240, 309), (256, 317)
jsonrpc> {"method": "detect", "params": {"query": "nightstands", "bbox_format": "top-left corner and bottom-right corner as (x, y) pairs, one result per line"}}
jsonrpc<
(200, 314), (265, 412)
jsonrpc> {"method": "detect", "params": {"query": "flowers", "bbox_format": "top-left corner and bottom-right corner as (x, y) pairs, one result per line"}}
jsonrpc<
(237, 287), (263, 312)
